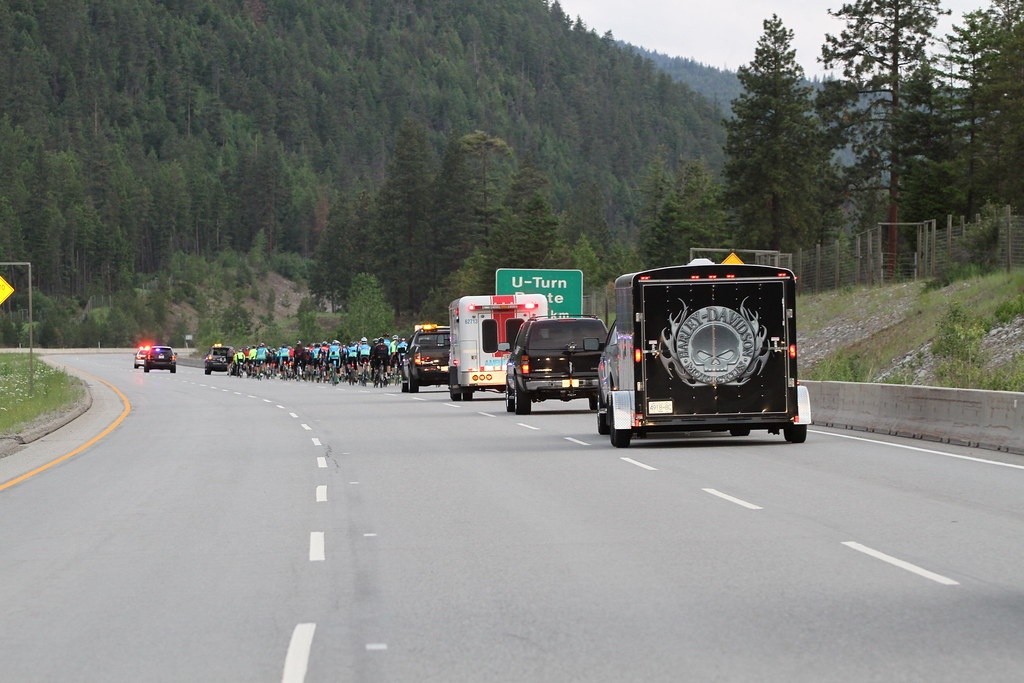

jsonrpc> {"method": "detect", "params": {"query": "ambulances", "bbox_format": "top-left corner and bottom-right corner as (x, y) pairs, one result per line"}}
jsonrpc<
(448, 294), (548, 401)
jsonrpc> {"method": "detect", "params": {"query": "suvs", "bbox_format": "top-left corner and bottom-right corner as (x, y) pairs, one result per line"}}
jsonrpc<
(398, 324), (450, 393)
(205, 343), (233, 374)
(144, 346), (177, 374)
(506, 314), (608, 415)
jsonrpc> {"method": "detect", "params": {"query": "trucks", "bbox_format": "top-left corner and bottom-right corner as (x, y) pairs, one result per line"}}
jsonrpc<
(583, 258), (812, 449)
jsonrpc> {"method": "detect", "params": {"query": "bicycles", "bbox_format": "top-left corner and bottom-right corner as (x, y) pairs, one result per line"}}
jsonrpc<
(235, 352), (406, 388)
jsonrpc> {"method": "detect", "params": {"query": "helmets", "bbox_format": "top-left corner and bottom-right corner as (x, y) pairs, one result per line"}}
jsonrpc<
(379, 337), (384, 343)
(237, 340), (359, 351)
(399, 336), (407, 341)
(361, 336), (367, 341)
(373, 338), (379, 343)
(392, 335), (399, 340)
(383, 333), (390, 337)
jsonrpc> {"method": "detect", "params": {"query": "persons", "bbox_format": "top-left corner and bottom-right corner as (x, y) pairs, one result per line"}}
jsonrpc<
(227, 335), (408, 385)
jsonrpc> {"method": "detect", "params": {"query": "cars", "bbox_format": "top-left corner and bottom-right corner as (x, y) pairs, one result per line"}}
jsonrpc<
(133, 347), (149, 368)
